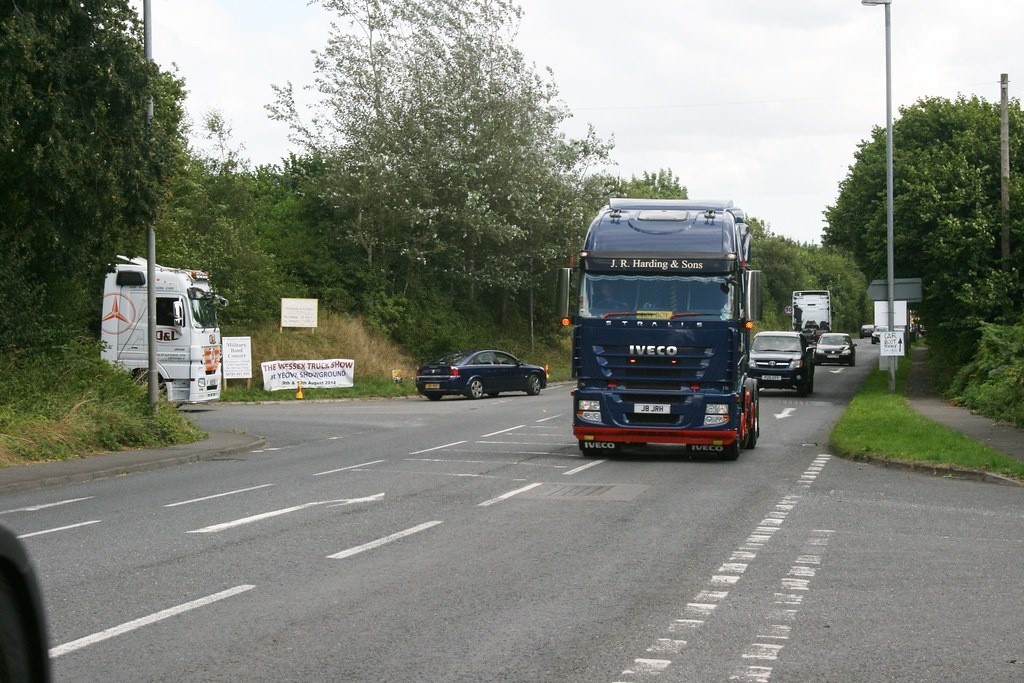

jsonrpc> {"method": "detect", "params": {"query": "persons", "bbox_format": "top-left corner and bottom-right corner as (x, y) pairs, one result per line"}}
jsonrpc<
(593, 280), (631, 313)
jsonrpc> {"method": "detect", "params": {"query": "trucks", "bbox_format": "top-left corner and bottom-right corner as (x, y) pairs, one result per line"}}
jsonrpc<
(97, 253), (230, 410)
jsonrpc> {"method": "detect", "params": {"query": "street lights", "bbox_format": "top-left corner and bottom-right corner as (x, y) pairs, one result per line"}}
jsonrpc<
(860, 0), (896, 393)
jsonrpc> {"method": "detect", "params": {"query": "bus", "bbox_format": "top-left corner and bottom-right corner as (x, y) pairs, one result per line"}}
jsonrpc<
(791, 289), (835, 336)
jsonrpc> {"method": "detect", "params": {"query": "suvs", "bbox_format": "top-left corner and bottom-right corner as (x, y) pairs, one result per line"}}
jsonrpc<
(859, 323), (875, 339)
(747, 330), (818, 397)
(871, 326), (889, 344)
(803, 327), (818, 344)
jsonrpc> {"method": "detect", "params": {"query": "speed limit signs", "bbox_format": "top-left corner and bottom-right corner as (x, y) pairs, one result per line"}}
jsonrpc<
(784, 305), (792, 314)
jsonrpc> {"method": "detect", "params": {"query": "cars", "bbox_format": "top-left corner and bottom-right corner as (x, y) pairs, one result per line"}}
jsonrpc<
(909, 321), (926, 343)
(415, 348), (548, 402)
(814, 332), (858, 367)
(813, 329), (829, 342)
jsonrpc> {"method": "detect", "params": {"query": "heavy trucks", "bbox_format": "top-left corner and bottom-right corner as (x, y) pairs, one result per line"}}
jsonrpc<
(556, 197), (759, 462)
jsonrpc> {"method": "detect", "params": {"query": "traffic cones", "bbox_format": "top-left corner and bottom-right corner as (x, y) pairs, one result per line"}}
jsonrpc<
(543, 364), (551, 379)
(295, 380), (305, 400)
